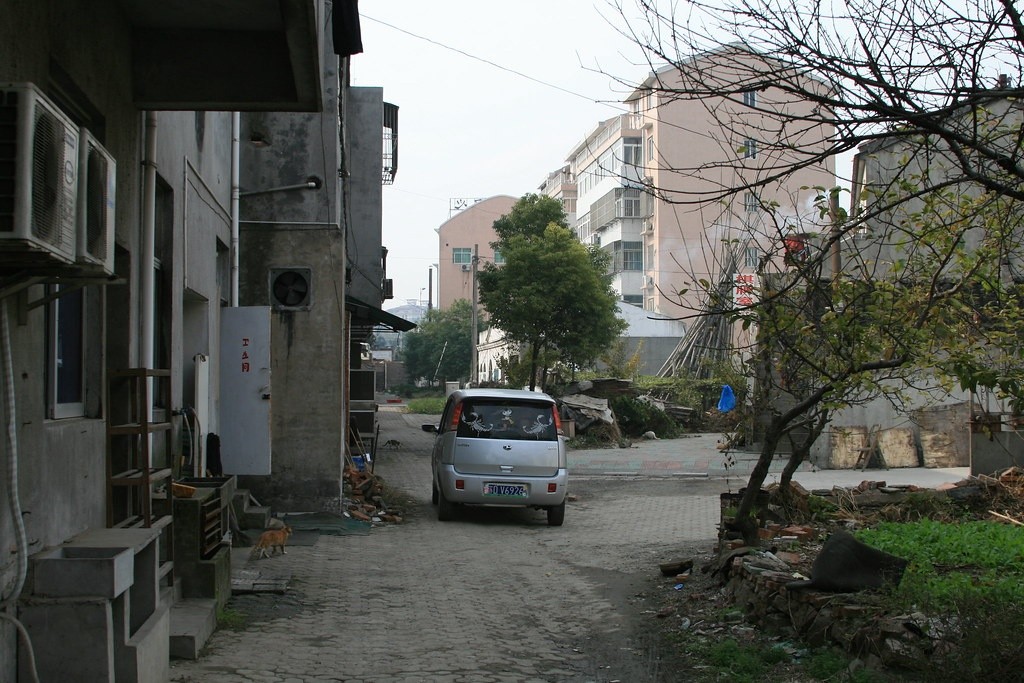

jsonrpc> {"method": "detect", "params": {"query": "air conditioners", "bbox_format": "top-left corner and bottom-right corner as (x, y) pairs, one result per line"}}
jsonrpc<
(0, 82), (81, 274)
(641, 275), (651, 287)
(461, 264), (470, 271)
(47, 126), (115, 275)
(590, 233), (598, 243)
(383, 278), (393, 297)
(641, 221), (651, 231)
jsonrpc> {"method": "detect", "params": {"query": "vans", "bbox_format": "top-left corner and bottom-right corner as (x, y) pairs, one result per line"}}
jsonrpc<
(422, 388), (567, 527)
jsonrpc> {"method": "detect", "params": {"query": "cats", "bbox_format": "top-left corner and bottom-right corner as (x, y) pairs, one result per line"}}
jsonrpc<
(247, 524), (292, 559)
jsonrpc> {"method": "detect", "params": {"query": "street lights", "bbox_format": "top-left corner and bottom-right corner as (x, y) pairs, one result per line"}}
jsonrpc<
(432, 263), (438, 310)
(420, 288), (425, 313)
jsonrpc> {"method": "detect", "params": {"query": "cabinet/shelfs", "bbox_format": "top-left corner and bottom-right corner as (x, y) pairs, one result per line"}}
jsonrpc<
(106, 367), (176, 586)
